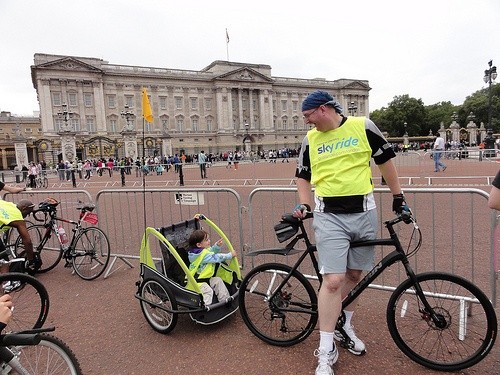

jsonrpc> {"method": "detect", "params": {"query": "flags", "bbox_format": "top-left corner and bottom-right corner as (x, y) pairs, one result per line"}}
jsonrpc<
(141, 88), (154, 125)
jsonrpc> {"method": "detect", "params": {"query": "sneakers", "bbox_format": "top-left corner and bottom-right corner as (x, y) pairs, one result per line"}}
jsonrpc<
(313, 341), (339, 375)
(333, 324), (367, 355)
(2, 280), (22, 294)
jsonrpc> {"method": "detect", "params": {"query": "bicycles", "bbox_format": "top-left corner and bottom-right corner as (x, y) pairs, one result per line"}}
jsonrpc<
(0, 197), (111, 281)
(238, 200), (498, 372)
(34, 171), (49, 189)
(0, 272), (84, 375)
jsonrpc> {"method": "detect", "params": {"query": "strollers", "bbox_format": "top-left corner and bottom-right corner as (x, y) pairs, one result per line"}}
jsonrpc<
(131, 212), (248, 334)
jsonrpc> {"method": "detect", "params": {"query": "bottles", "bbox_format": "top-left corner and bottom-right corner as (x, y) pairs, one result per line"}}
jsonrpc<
(58, 226), (68, 244)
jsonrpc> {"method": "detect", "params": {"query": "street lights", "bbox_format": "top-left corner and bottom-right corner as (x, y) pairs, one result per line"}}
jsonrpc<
(482, 59), (498, 135)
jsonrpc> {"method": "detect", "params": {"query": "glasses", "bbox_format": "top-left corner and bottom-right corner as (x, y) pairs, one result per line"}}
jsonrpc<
(302, 107), (318, 119)
(25, 203), (36, 208)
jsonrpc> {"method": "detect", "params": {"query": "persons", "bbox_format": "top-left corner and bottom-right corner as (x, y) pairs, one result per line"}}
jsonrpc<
(184, 229), (237, 306)
(390, 130), (489, 173)
(0, 294), (14, 336)
(294, 91), (413, 375)
(0, 181), (26, 196)
(487, 170), (500, 211)
(50, 146), (299, 181)
(14, 159), (47, 189)
(1, 199), (37, 294)
